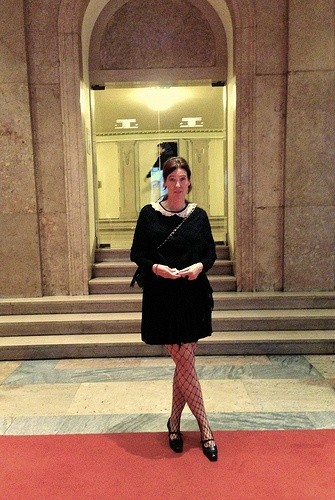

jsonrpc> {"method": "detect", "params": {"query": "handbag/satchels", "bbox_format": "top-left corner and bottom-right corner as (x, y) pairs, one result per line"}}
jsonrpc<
(131, 252), (168, 287)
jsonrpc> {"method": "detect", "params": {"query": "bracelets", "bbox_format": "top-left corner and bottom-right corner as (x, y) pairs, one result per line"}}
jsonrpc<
(154, 263), (160, 276)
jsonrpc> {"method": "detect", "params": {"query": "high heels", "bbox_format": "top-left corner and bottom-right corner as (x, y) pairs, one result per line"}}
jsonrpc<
(167, 418), (184, 453)
(200, 430), (218, 462)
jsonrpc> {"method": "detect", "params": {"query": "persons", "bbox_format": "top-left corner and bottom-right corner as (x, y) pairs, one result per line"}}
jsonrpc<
(129, 156), (221, 461)
